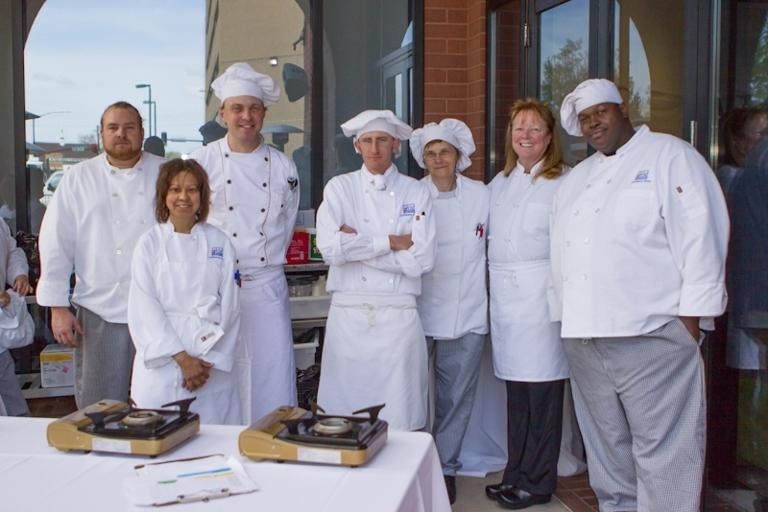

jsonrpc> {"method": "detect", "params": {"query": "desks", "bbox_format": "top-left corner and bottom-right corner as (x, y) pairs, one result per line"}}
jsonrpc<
(0, 416), (455, 512)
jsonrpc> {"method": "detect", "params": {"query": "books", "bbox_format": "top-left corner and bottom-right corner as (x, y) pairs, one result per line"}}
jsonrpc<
(133, 453), (262, 506)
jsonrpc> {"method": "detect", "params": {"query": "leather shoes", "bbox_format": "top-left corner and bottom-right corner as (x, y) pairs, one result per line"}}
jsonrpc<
(485, 482), (513, 501)
(496, 486), (552, 510)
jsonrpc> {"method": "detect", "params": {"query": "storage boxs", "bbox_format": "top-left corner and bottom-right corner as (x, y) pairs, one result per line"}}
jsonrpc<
(39, 343), (75, 386)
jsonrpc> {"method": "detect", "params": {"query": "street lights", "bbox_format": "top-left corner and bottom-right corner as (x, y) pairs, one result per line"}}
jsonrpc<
(135, 83), (152, 137)
(143, 100), (156, 136)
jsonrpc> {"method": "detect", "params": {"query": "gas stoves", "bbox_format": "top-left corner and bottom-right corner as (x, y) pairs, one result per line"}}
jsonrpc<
(238, 403), (389, 469)
(46, 395), (201, 458)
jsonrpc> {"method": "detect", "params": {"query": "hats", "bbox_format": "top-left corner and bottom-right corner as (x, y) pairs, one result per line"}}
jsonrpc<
(209, 62), (282, 109)
(559, 78), (624, 137)
(0, 291), (38, 350)
(339, 109), (413, 143)
(409, 117), (476, 172)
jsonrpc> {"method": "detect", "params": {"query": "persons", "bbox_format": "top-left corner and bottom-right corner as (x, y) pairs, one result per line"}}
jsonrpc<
(35, 102), (170, 410)
(183, 62), (300, 424)
(409, 118), (491, 506)
(323, 133), (362, 186)
(548, 79), (731, 512)
(727, 133), (768, 488)
(143, 136), (166, 158)
(316, 109), (438, 433)
(712, 103), (767, 490)
(126, 158), (242, 425)
(27, 149), (45, 230)
(0, 215), (35, 417)
(198, 120), (227, 147)
(485, 98), (575, 510)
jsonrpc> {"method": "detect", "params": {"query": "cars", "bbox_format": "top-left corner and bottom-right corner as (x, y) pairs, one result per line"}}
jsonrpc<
(38, 169), (67, 205)
